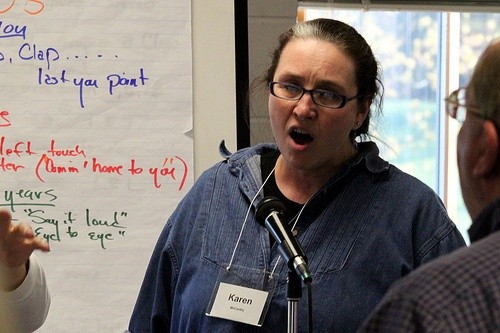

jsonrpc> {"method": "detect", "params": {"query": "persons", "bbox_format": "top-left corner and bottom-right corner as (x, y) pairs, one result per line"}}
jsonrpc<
(0, 210), (51, 332)
(127, 18), (467, 333)
(355, 36), (500, 333)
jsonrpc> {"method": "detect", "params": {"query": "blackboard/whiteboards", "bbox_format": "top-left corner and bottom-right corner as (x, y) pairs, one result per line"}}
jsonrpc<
(0, 0), (251, 333)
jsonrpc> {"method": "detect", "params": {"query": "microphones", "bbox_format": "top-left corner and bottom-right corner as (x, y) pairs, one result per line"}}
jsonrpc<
(254, 196), (312, 284)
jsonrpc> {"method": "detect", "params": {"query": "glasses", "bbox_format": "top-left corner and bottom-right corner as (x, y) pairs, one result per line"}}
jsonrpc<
(445, 88), (499, 127)
(269, 79), (361, 109)
